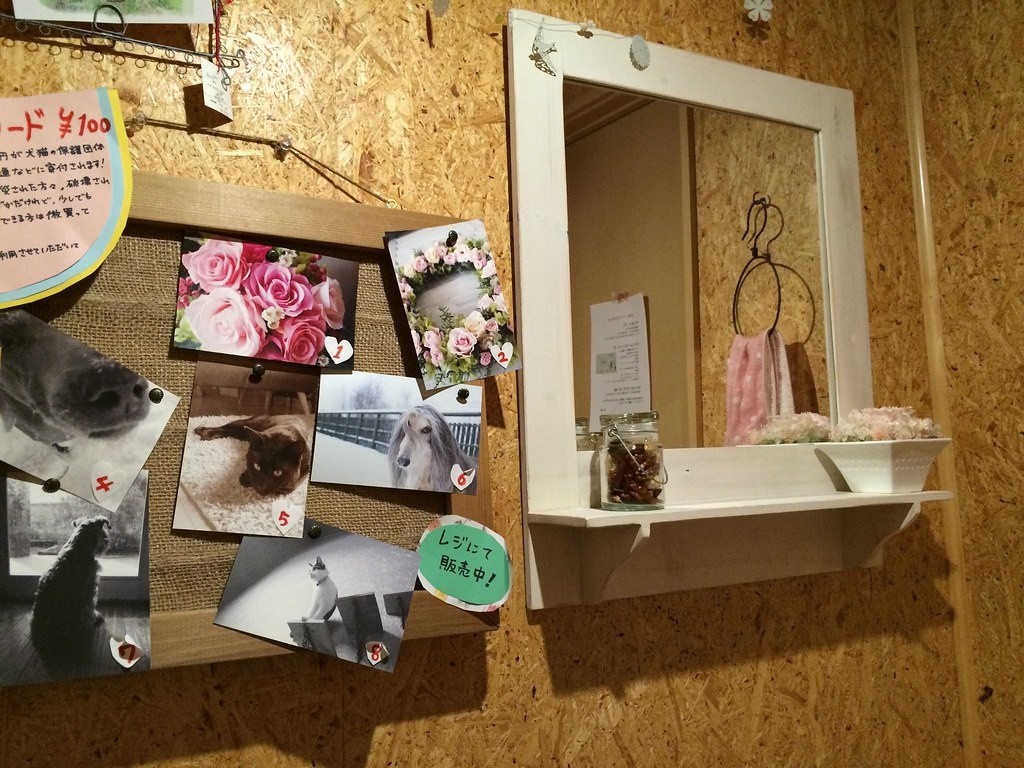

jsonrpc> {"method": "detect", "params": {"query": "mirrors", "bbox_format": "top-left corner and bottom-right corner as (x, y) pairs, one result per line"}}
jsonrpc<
(507, 9), (952, 609)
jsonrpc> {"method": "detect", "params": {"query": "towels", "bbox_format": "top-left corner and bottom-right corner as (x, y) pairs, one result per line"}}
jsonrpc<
(723, 324), (797, 448)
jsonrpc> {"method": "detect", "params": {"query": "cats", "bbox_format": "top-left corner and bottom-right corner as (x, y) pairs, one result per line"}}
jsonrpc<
(192, 414), (312, 496)
(301, 556), (338, 621)
(0, 309), (149, 447)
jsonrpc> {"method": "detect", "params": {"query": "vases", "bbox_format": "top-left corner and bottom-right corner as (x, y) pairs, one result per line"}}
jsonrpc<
(814, 437), (952, 491)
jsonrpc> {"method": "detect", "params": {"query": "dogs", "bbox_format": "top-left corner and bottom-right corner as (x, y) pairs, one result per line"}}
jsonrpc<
(387, 404), (477, 494)
(30, 515), (112, 658)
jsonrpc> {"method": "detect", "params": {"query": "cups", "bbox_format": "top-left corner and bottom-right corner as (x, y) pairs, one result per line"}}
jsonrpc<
(576, 418), (600, 451)
(600, 412), (665, 510)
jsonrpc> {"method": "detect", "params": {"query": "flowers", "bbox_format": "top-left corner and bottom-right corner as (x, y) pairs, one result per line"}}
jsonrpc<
(829, 405), (942, 442)
(535, 20), (557, 74)
(735, 411), (830, 445)
(577, 18), (596, 34)
(743, 0), (774, 22)
(631, 35), (651, 69)
